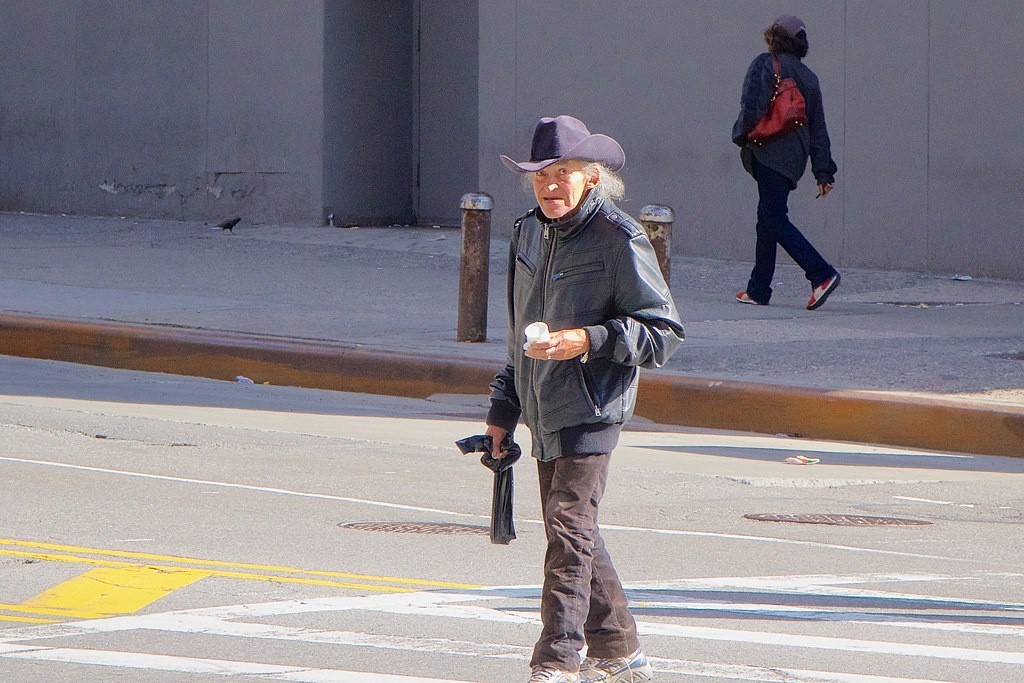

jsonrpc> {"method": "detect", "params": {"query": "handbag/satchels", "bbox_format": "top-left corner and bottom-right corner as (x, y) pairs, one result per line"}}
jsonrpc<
(747, 54), (809, 148)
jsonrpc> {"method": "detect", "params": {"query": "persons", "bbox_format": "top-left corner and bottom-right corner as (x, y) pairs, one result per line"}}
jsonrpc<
(484, 114), (688, 682)
(730, 15), (841, 311)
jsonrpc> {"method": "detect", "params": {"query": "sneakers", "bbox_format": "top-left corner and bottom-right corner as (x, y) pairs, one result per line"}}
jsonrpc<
(579, 647), (652, 682)
(527, 664), (580, 683)
(807, 272), (841, 311)
(736, 291), (769, 306)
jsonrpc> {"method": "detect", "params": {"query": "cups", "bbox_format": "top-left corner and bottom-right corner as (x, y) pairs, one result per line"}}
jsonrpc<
(524, 321), (550, 342)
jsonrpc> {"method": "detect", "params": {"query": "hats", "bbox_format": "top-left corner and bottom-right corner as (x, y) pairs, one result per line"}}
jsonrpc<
(761, 14), (806, 37)
(498, 114), (626, 175)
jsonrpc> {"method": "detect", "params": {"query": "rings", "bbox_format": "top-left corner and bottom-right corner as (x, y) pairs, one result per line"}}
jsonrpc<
(547, 355), (552, 361)
